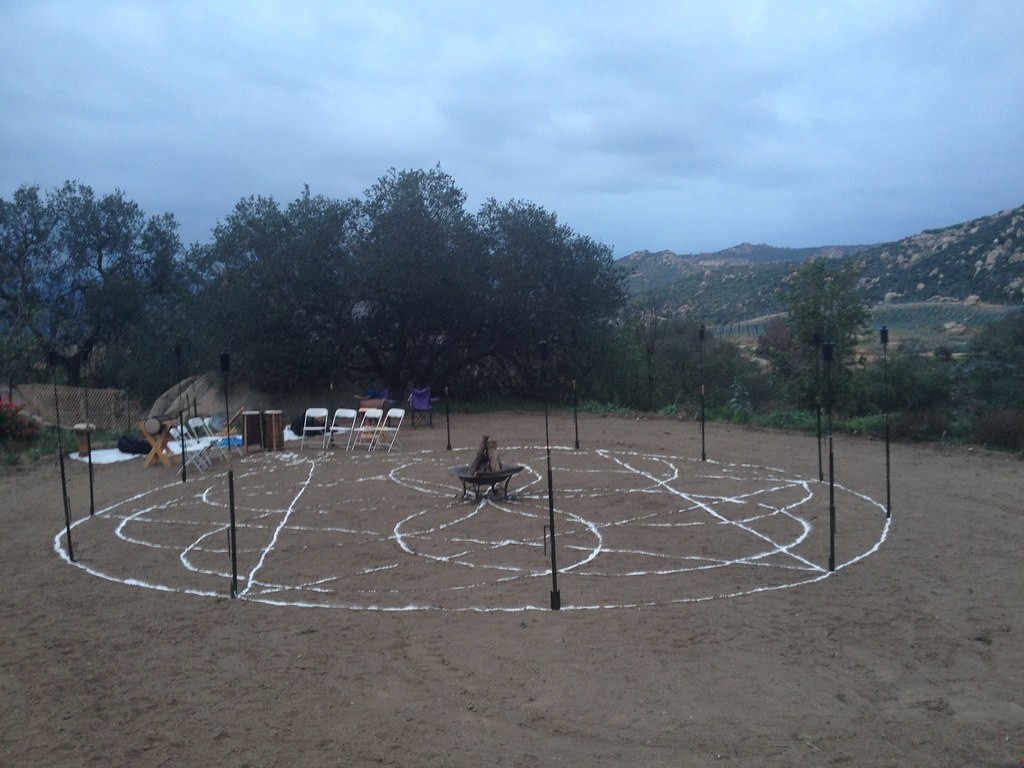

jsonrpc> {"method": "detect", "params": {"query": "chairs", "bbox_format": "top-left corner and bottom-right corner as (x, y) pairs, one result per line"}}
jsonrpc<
(167, 417), (241, 477)
(301, 387), (433, 452)
